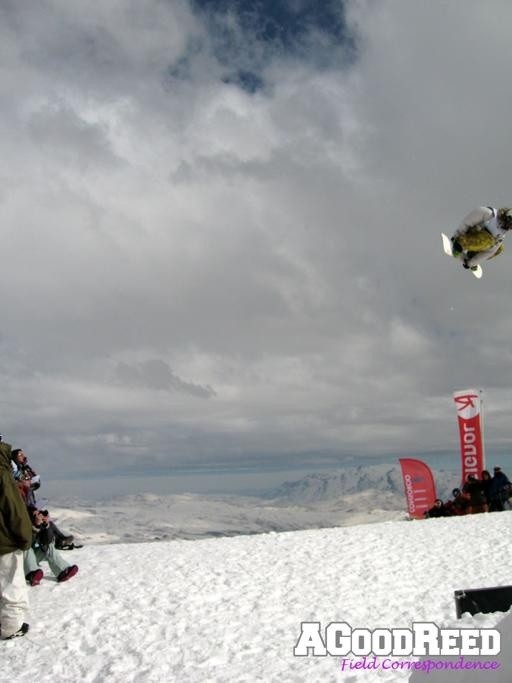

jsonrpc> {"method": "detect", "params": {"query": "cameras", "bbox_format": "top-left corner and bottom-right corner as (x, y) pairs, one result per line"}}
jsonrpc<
(39, 509), (49, 517)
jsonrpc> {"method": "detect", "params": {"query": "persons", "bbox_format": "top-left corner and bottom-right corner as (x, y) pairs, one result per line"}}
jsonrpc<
(419, 464), (512, 516)
(22, 503), (80, 586)
(36, 504), (76, 552)
(449, 204), (511, 271)
(11, 449), (44, 502)
(1, 440), (30, 641)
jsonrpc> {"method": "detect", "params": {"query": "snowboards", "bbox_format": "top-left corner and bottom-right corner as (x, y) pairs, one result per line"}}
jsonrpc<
(441, 231), (484, 279)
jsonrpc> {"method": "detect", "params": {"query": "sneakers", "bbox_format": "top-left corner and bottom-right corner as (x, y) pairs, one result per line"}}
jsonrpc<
(56, 565), (79, 582)
(29, 569), (43, 586)
(2, 622), (29, 641)
(62, 535), (73, 545)
